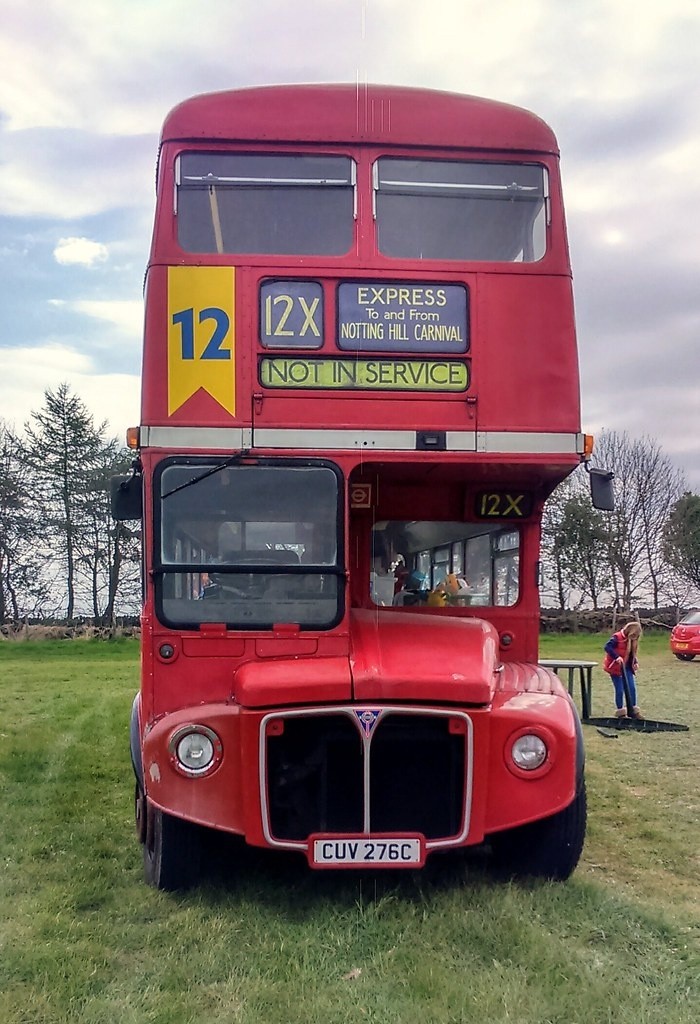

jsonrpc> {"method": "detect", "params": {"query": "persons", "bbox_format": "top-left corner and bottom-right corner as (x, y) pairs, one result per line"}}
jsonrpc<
(603, 622), (645, 721)
(388, 560), (468, 607)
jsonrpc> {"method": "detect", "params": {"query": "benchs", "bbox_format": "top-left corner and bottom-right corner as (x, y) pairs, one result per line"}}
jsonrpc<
(221, 549), (300, 564)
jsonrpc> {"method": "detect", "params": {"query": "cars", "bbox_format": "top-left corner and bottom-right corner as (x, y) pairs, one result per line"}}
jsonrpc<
(670, 608), (700, 661)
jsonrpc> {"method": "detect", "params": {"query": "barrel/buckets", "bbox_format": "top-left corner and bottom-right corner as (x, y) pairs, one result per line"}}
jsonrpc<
(370, 572), (398, 607)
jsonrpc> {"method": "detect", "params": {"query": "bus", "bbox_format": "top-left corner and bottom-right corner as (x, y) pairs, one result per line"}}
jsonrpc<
(110, 82), (616, 893)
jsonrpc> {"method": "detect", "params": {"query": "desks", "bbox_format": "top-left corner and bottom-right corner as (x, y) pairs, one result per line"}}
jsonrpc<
(538, 660), (599, 719)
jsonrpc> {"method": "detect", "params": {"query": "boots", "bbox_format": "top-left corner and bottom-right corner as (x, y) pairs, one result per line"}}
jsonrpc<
(615, 707), (632, 718)
(632, 706), (645, 719)
